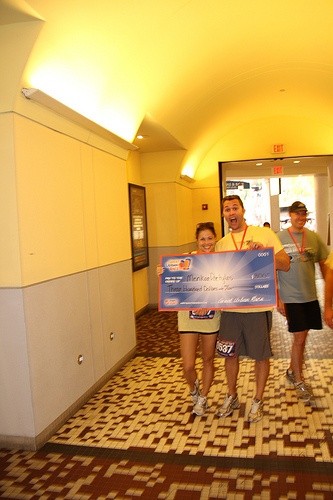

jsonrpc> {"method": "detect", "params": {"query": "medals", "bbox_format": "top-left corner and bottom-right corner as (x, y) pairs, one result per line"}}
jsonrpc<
(300, 256), (306, 262)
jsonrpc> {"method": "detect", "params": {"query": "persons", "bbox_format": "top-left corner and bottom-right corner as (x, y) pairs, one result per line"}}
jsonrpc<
(275, 201), (330, 401)
(215, 195), (291, 423)
(156, 222), (221, 417)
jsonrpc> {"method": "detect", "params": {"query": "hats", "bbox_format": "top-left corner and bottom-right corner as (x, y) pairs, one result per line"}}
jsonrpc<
(290, 201), (308, 212)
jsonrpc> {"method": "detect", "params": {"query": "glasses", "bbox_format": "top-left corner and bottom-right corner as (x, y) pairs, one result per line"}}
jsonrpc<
(196, 221), (213, 231)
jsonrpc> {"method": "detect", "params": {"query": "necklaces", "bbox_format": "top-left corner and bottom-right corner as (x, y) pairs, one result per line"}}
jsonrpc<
(231, 226), (248, 250)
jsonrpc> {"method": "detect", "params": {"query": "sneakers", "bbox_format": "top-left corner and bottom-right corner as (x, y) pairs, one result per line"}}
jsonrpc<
(248, 398), (264, 422)
(189, 378), (200, 403)
(296, 382), (311, 403)
(192, 396), (208, 416)
(215, 393), (240, 417)
(285, 368), (305, 386)
